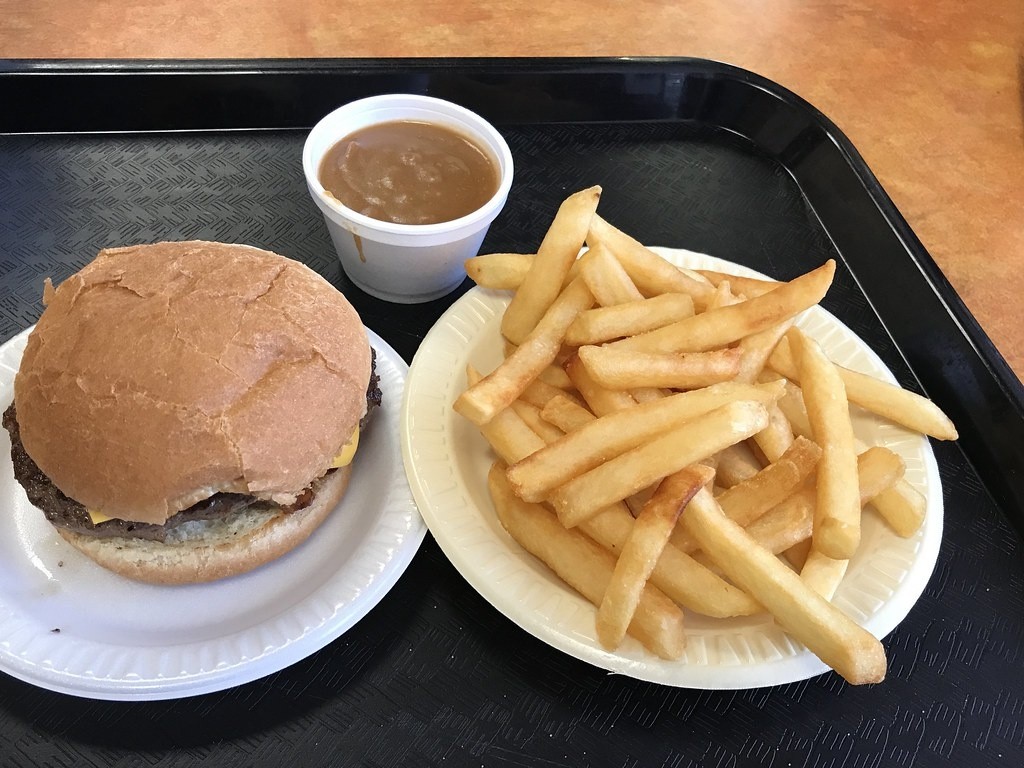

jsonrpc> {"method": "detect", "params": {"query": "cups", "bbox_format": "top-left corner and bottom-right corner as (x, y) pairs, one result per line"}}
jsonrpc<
(304, 92), (517, 305)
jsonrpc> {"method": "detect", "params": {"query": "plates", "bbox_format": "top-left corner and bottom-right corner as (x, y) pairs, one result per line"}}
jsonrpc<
(399, 245), (947, 689)
(0, 323), (428, 701)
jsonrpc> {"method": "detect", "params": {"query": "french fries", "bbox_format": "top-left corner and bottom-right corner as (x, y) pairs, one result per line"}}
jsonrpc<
(455, 183), (959, 686)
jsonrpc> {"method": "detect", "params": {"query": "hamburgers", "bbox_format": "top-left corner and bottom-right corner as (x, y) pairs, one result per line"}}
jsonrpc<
(3, 238), (380, 585)
